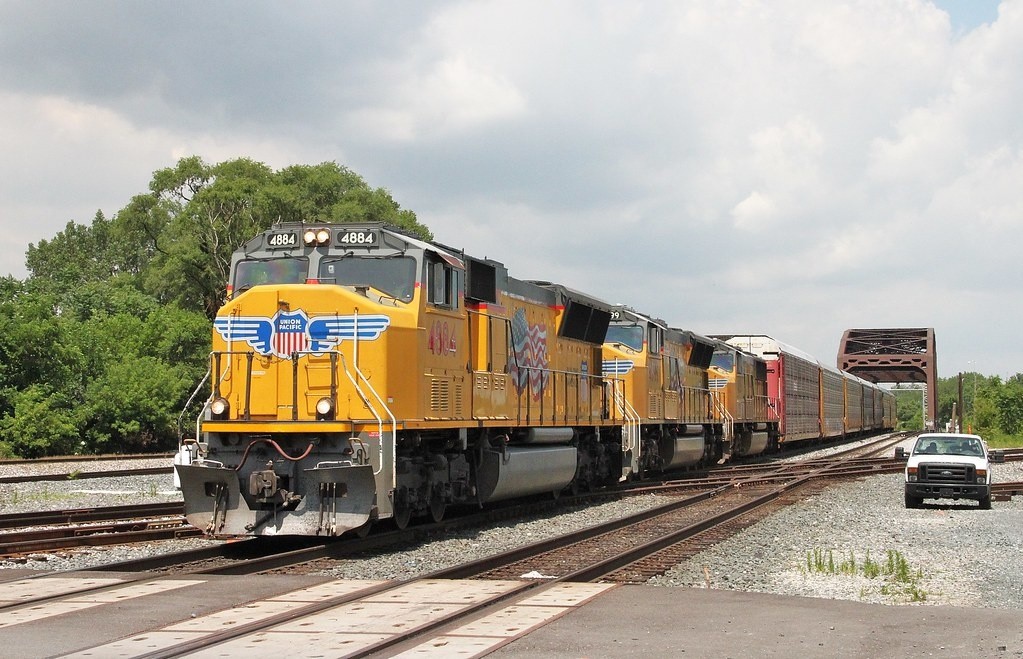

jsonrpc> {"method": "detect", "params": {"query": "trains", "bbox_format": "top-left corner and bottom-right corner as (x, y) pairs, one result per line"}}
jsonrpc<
(175, 216), (898, 542)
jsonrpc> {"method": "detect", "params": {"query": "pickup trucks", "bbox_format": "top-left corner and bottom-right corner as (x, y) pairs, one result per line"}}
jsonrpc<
(895, 433), (1006, 509)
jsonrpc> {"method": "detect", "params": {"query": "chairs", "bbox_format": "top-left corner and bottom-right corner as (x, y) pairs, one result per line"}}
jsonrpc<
(962, 442), (969, 450)
(926, 442), (937, 453)
